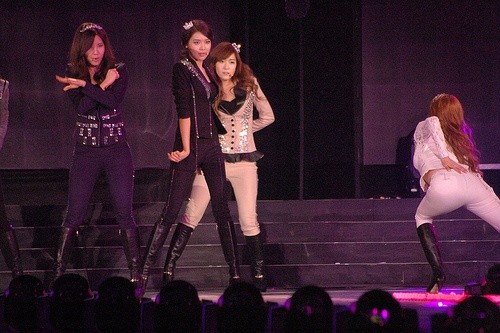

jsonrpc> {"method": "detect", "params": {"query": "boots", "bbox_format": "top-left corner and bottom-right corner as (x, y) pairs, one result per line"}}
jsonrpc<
(118, 226), (142, 288)
(48, 226), (75, 288)
(245, 230), (267, 292)
(0, 229), (23, 278)
(417, 222), (445, 294)
(161, 222), (194, 292)
(138, 223), (171, 293)
(216, 220), (240, 286)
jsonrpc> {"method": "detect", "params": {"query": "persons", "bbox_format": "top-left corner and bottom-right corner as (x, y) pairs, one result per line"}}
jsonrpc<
(135, 19), (240, 294)
(157, 41), (276, 291)
(46, 24), (143, 290)
(0, 77), (25, 274)
(412, 93), (500, 293)
